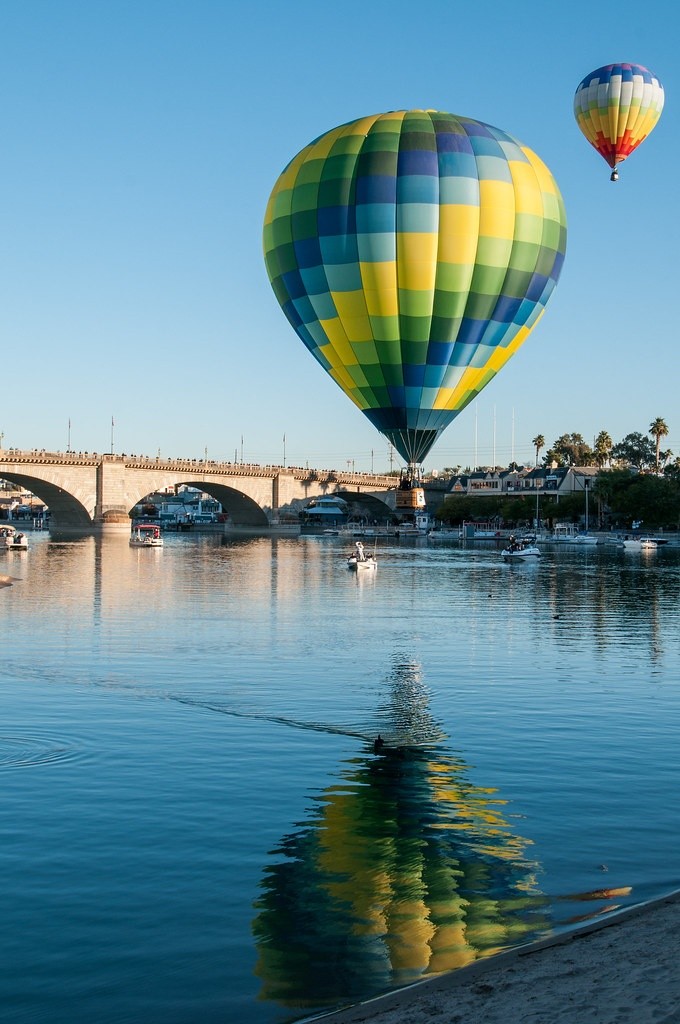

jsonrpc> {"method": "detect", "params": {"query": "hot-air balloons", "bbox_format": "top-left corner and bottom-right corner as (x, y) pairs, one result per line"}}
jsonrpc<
(573, 62), (667, 181)
(261, 109), (570, 510)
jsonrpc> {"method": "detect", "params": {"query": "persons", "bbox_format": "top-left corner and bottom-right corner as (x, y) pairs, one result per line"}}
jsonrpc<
(121, 451), (400, 482)
(350, 553), (357, 558)
(506, 535), (535, 554)
(9, 446), (98, 460)
(154, 528), (158, 538)
(399, 476), (420, 489)
(0, 528), (24, 544)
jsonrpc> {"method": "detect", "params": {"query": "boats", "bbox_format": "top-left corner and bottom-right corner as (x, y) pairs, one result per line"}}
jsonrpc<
(501, 536), (541, 560)
(323, 523), (427, 537)
(0, 525), (30, 550)
(623, 540), (657, 549)
(536, 523), (606, 545)
(347, 542), (377, 571)
(129, 524), (163, 548)
(607, 529), (670, 545)
